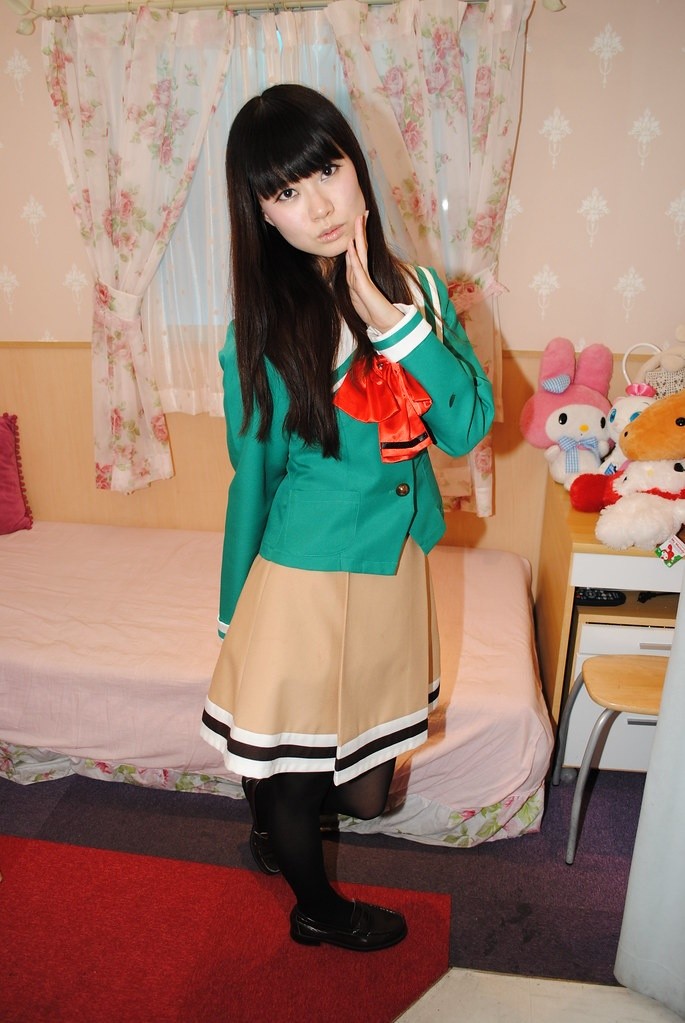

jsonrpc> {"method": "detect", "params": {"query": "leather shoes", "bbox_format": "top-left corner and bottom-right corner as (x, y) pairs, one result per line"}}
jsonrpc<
(289, 900), (407, 949)
(242, 776), (281, 875)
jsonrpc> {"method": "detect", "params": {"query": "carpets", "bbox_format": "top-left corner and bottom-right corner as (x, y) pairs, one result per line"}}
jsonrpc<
(0, 836), (451, 1023)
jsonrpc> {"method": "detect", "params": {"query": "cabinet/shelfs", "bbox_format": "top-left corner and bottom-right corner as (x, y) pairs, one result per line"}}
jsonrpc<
(558, 593), (679, 783)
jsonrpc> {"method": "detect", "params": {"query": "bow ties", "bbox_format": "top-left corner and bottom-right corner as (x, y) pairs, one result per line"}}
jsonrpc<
(332, 352), (434, 463)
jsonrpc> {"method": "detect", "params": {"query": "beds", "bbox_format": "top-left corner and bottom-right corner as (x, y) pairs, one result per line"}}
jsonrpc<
(0, 412), (555, 849)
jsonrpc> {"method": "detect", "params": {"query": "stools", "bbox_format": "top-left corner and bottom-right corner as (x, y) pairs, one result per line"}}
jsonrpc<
(550, 655), (668, 866)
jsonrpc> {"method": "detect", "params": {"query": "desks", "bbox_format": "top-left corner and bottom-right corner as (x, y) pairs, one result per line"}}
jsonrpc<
(533, 463), (685, 774)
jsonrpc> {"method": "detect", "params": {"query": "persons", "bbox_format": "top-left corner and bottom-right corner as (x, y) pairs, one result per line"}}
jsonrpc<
(201, 84), (496, 952)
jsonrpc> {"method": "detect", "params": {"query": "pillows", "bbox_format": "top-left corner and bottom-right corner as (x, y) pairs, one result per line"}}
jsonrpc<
(0, 413), (34, 536)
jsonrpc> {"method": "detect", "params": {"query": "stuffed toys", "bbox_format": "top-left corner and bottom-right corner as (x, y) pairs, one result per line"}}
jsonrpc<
(519, 338), (613, 484)
(570, 326), (685, 550)
